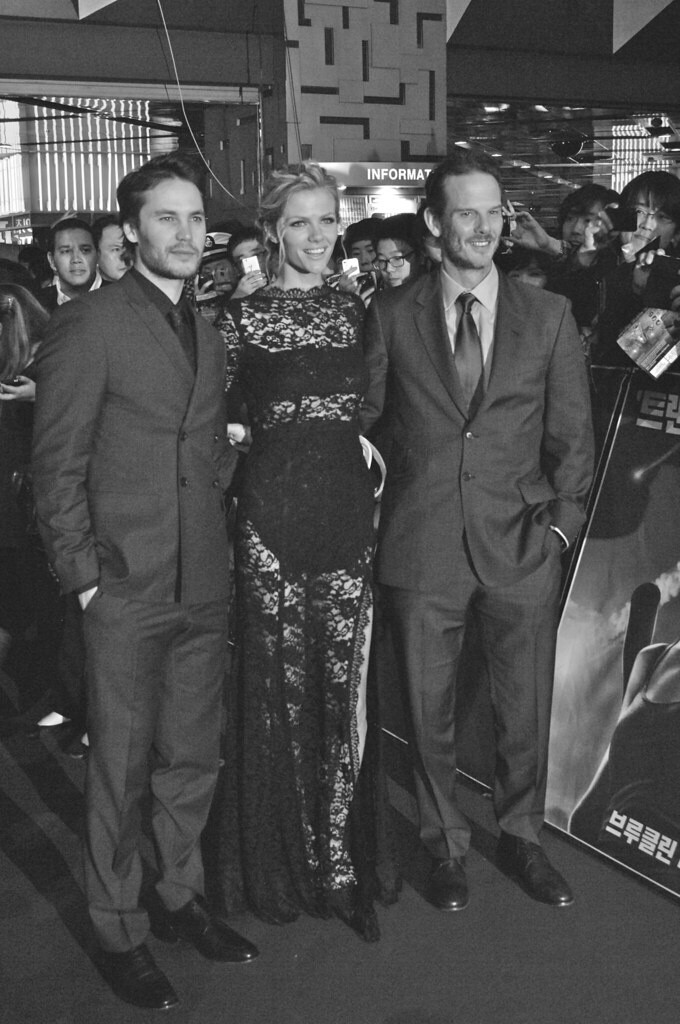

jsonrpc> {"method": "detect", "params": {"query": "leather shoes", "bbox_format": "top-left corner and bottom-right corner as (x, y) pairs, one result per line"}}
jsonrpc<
(150, 899), (260, 965)
(38, 704), (94, 746)
(419, 851), (470, 915)
(90, 944), (180, 1011)
(495, 829), (572, 905)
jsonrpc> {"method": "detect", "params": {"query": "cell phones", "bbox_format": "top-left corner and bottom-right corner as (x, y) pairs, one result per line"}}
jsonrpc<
(342, 258), (362, 288)
(196, 271), (215, 293)
(604, 207), (637, 232)
(356, 273), (377, 299)
(242, 255), (263, 281)
(641, 254), (680, 308)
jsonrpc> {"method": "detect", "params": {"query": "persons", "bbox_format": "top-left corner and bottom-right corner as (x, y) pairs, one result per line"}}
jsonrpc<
(496, 170), (679, 373)
(190, 221), (272, 315)
(360, 157), (595, 913)
(36, 150), (260, 1010)
(569, 641), (680, 896)
(0, 212), (133, 754)
(320, 209), (427, 313)
(217, 164), (407, 941)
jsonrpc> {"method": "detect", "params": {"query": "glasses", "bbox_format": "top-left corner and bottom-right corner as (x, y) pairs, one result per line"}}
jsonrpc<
(372, 252), (420, 266)
(631, 205), (674, 223)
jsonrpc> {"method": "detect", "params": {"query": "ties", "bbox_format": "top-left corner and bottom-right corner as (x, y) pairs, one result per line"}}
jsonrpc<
(453, 294), (487, 409)
(172, 312), (202, 366)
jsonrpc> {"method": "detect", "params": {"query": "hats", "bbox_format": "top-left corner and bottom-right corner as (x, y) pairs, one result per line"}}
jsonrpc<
(199, 232), (231, 260)
